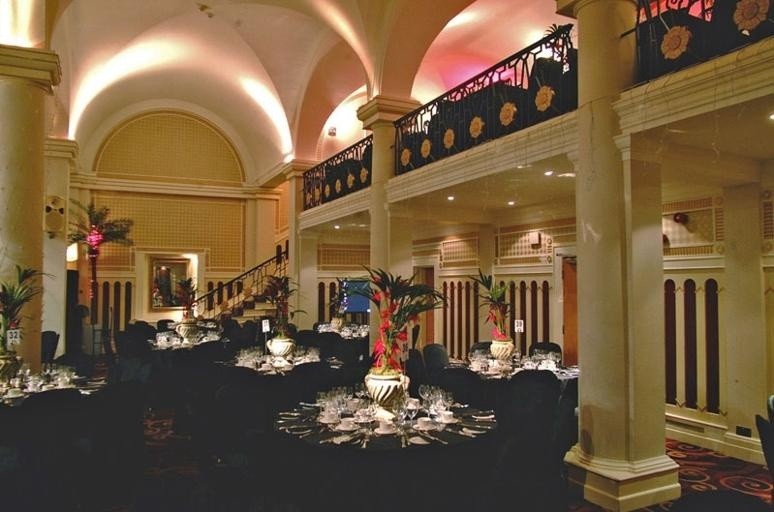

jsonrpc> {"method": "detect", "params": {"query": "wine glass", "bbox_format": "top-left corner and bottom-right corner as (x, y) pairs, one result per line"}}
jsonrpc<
(317, 382), (455, 437)
(240, 342), (320, 371)
(320, 322), (370, 340)
(0, 362), (78, 389)
(154, 331), (222, 346)
(463, 349), (572, 376)
(167, 321), (217, 329)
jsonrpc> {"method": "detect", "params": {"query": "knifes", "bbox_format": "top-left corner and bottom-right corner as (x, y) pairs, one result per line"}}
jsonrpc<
(278, 398), (315, 440)
(318, 433), (449, 448)
(458, 408), (495, 438)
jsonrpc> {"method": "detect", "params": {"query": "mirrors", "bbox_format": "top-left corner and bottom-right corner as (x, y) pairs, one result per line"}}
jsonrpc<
(146, 253), (191, 314)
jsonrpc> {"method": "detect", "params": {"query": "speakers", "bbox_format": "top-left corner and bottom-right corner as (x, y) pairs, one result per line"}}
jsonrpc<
(528, 232), (540, 244)
(43, 195), (65, 233)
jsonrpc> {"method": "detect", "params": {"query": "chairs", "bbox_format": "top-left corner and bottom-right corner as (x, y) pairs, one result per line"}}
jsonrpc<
(0, 318), (580, 511)
(525, 0), (774, 130)
(325, 79), (527, 203)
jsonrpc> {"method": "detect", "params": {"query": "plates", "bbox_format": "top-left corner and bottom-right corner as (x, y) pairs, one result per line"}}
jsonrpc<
(3, 394), (24, 400)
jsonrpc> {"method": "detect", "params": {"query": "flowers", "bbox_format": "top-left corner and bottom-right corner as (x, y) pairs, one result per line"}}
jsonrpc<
(173, 277), (198, 309)
(0, 265), (56, 356)
(259, 272), (309, 337)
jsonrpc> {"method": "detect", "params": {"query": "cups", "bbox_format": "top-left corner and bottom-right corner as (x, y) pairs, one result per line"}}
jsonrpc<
(8, 389), (20, 395)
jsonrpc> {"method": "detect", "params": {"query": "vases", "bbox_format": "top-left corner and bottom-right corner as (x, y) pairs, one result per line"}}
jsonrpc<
(466, 268), (515, 340)
(175, 309), (199, 339)
(330, 264), (451, 375)
